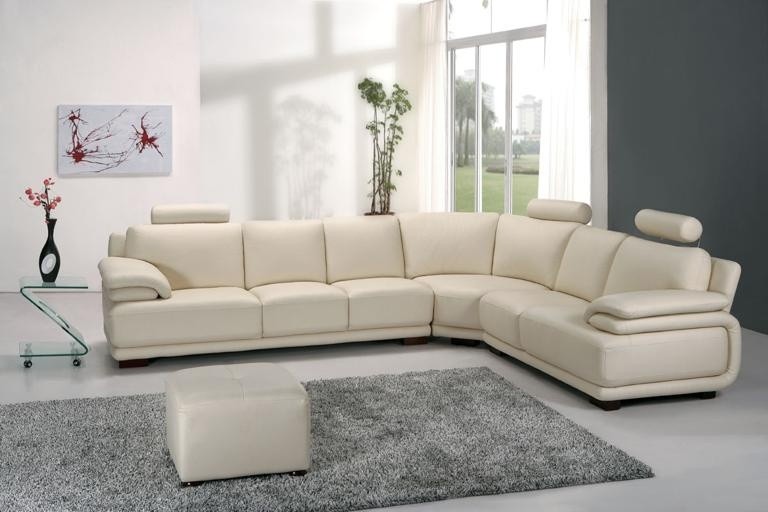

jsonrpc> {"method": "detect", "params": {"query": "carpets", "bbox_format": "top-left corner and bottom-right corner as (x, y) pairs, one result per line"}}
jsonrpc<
(1, 365), (655, 512)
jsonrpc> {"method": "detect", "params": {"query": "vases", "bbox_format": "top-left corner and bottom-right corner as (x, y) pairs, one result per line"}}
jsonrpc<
(38, 218), (61, 283)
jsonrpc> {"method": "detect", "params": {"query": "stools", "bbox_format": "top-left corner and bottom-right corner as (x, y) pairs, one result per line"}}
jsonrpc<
(165, 361), (311, 485)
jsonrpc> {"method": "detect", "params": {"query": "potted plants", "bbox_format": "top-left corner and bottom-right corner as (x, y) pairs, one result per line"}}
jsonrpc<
(358, 77), (412, 216)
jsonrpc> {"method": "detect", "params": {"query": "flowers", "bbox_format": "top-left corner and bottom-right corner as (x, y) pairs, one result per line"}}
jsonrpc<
(19, 176), (62, 225)
